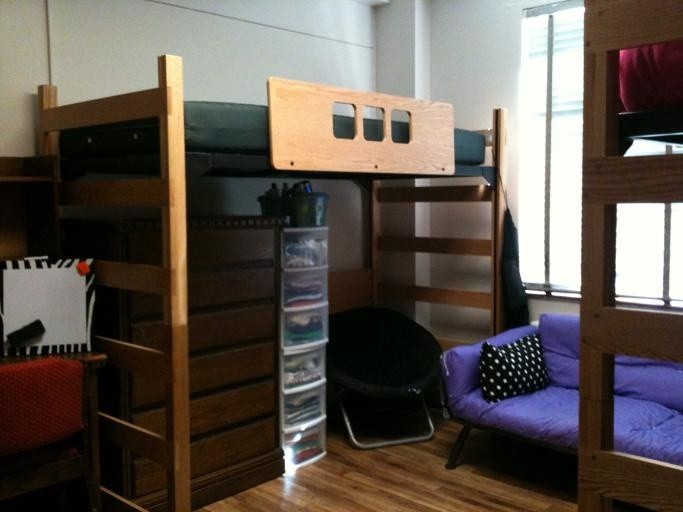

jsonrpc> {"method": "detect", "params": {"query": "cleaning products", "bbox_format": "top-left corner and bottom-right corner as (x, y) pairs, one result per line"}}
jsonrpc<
(265, 180), (312, 198)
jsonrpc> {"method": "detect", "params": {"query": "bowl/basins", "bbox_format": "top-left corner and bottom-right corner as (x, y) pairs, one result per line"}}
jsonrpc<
(261, 192), (330, 227)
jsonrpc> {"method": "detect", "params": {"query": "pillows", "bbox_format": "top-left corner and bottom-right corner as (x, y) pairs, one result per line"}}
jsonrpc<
(480, 331), (549, 405)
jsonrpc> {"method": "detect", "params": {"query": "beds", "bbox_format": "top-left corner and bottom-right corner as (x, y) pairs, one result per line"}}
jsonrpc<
(576, 0), (683, 512)
(37, 54), (505, 510)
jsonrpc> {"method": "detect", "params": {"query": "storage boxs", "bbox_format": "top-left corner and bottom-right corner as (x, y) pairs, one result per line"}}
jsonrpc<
(257, 194), (329, 473)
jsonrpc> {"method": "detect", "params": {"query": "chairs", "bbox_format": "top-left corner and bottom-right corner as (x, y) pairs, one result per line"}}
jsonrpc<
(0, 353), (100, 512)
(327, 306), (442, 450)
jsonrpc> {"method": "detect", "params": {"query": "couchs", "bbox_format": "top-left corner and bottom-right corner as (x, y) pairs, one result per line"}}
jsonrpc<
(440, 313), (682, 470)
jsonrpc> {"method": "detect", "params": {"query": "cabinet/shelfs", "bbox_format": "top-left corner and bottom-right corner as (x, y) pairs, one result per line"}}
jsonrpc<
(60, 215), (285, 512)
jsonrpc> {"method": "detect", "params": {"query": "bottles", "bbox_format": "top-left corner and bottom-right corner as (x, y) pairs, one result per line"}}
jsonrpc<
(267, 182), (289, 198)
